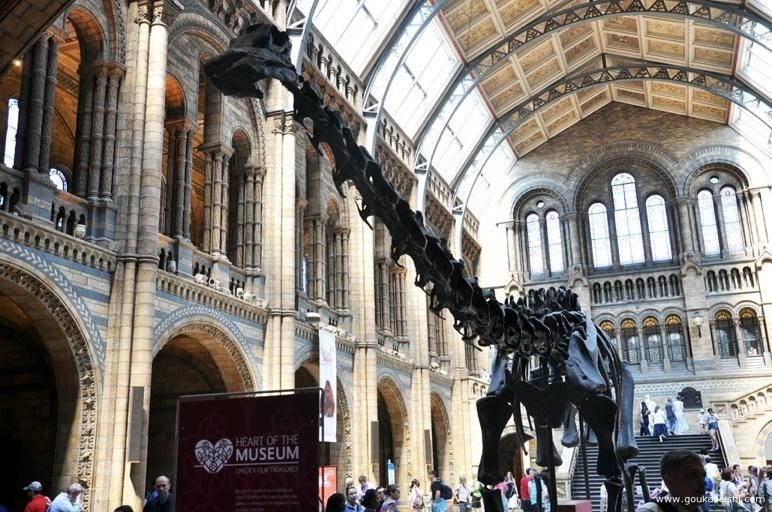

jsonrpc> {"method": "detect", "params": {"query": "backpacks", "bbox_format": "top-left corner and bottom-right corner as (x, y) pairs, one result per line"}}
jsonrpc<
(504, 483), (514, 498)
(435, 482), (451, 499)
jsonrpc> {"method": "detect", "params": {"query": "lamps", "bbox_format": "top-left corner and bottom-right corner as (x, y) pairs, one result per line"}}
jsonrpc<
(710, 174), (719, 185)
(536, 200), (544, 208)
(693, 312), (703, 340)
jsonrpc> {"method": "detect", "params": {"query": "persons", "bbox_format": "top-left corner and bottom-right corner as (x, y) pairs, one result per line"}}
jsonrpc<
(639, 393), (689, 443)
(695, 409), (707, 434)
(0, 474), (175, 512)
(325, 468), (567, 512)
(634, 450), (772, 512)
(705, 408), (720, 452)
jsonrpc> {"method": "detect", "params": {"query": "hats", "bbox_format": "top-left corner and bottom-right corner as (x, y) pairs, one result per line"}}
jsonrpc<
(24, 482), (41, 491)
